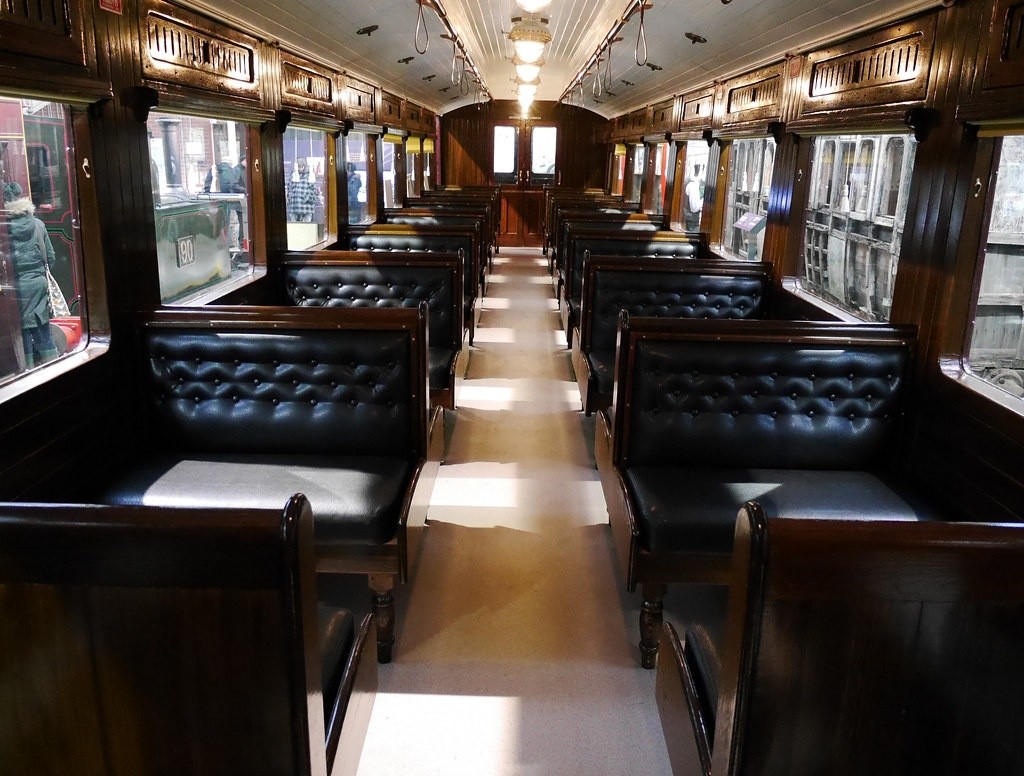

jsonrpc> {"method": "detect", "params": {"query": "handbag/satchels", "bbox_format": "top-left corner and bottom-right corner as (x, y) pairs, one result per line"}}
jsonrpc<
(45, 263), (71, 318)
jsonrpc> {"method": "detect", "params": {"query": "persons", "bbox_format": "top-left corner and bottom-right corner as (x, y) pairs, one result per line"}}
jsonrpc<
(685, 176), (704, 228)
(345, 162), (362, 222)
(0, 180), (58, 370)
(204, 154), (247, 255)
(287, 156), (328, 243)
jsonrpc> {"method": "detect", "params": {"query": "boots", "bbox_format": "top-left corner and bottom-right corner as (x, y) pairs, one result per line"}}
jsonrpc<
(25, 353), (35, 369)
(40, 347), (59, 364)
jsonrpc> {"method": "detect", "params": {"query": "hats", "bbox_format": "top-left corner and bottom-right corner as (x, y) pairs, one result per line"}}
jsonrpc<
(1, 181), (22, 203)
(220, 155), (233, 164)
(347, 162), (356, 172)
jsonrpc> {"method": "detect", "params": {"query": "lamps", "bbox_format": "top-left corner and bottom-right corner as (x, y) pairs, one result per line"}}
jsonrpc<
(502, 0), (553, 114)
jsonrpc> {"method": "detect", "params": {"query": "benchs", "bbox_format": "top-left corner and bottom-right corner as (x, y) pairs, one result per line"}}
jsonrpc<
(541, 184), (1024, 776)
(0, 187), (503, 776)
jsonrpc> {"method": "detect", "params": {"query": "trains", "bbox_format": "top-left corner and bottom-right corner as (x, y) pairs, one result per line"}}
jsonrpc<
(631, 132), (1024, 400)
